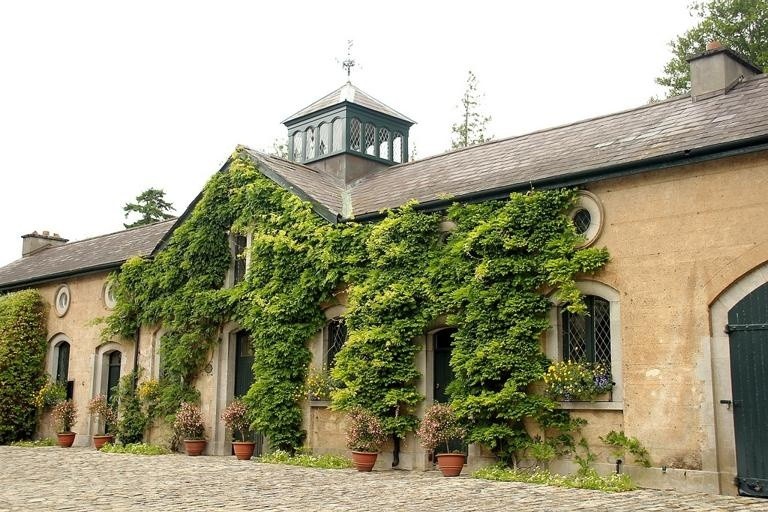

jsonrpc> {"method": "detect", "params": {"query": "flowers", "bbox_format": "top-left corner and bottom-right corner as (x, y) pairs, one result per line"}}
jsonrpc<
(220, 395), (254, 442)
(415, 400), (467, 452)
(344, 407), (389, 452)
(542, 358), (616, 401)
(175, 399), (207, 440)
(51, 400), (79, 433)
(87, 395), (117, 435)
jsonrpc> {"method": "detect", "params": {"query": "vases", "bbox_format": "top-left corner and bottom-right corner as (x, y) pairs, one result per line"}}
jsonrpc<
(352, 451), (377, 472)
(436, 454), (466, 477)
(57, 434), (76, 448)
(232, 442), (255, 460)
(93, 436), (112, 450)
(184, 440), (207, 456)
